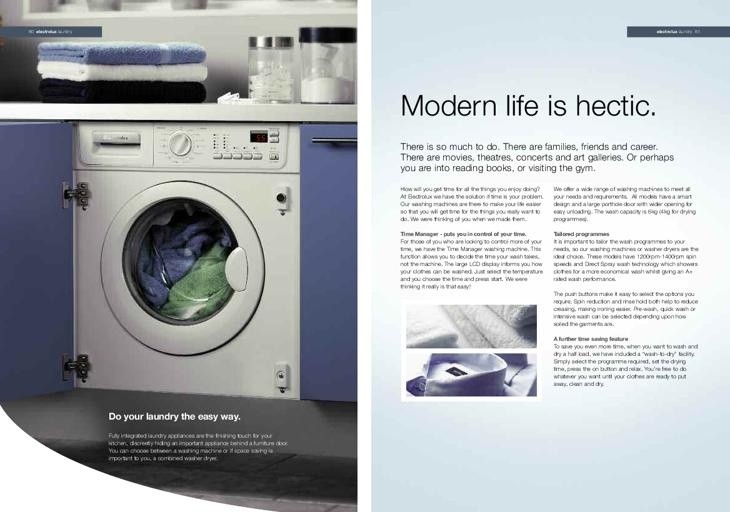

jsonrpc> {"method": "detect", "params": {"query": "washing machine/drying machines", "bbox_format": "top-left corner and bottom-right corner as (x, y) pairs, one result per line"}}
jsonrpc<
(75, 121), (299, 400)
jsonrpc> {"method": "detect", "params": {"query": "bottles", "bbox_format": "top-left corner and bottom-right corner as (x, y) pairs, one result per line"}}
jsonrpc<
(246, 34), (296, 104)
(299, 25), (357, 104)
